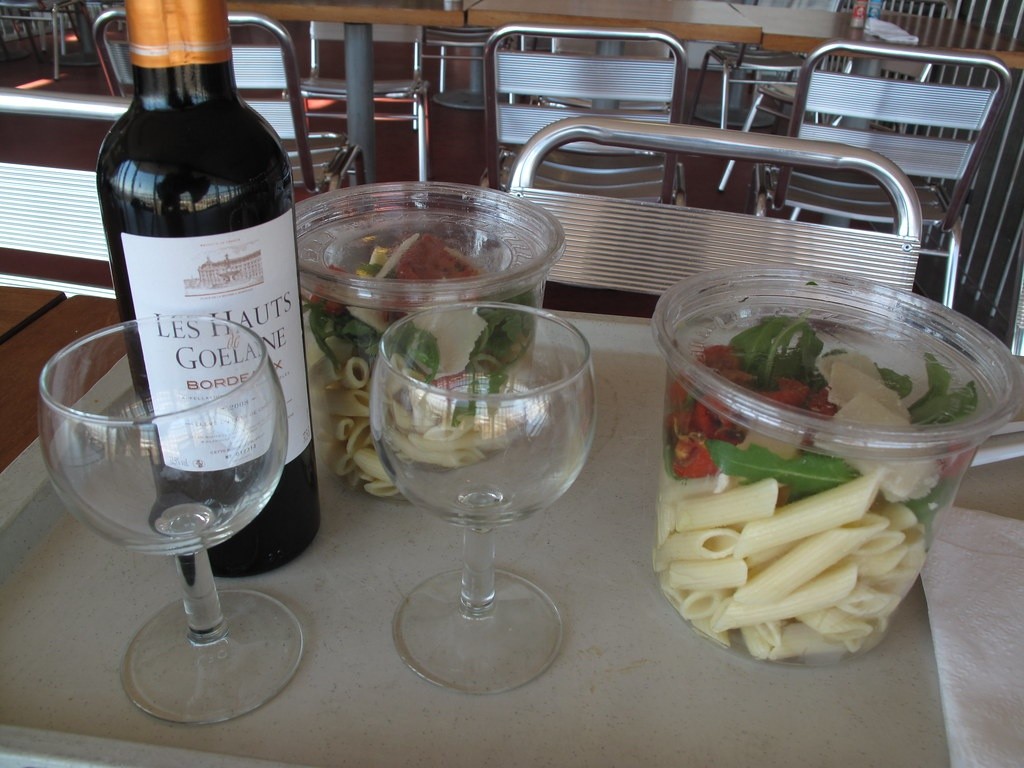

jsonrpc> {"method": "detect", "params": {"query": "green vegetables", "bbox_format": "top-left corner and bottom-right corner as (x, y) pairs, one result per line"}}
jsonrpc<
(299, 263), (540, 426)
(695, 315), (977, 524)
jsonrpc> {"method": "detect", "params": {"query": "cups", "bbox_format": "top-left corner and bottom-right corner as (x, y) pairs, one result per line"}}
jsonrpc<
(648, 267), (1024, 663)
(293, 181), (565, 504)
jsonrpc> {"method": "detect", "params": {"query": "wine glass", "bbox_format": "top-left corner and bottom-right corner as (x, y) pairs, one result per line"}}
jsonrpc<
(371, 301), (599, 700)
(36, 317), (308, 727)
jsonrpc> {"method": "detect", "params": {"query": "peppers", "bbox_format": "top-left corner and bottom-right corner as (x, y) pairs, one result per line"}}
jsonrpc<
(664, 344), (836, 479)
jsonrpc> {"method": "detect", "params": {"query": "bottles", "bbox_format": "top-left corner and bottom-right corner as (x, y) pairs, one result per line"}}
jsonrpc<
(94, 0), (322, 579)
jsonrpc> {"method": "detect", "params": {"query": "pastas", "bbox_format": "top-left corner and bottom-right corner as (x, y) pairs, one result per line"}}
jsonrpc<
(655, 467), (926, 660)
(315, 356), (527, 498)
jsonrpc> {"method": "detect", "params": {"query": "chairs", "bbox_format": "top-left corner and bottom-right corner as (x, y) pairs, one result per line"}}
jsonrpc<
(91, 0), (1014, 311)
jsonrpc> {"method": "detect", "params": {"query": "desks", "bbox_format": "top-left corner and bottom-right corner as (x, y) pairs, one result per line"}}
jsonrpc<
(730, 0), (1024, 280)
(227, 0), (481, 190)
(467, 0), (763, 165)
(0, 287), (1024, 768)
